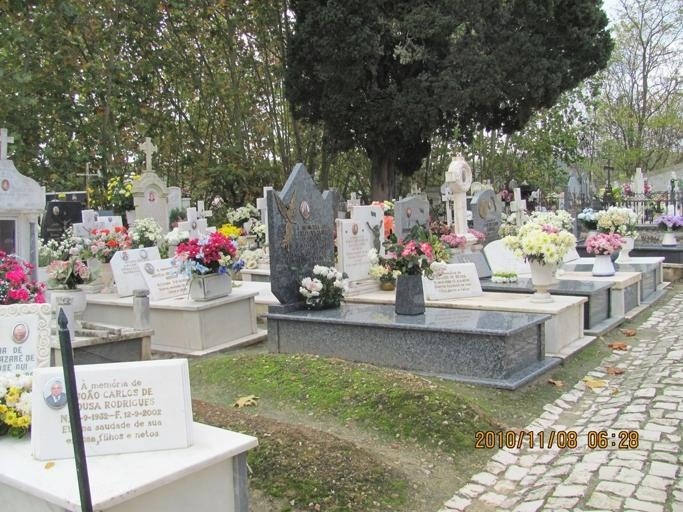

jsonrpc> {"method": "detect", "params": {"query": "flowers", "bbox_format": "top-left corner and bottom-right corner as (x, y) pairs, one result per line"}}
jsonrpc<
(128, 218), (170, 259)
(361, 216), (456, 284)
(372, 200), (397, 216)
(0, 251), (46, 307)
(429, 220), (485, 249)
(37, 226), (130, 293)
(167, 202), (270, 275)
(497, 209), (577, 267)
(492, 271), (518, 284)
(577, 205), (641, 256)
(653, 213), (681, 233)
(299, 264), (349, 310)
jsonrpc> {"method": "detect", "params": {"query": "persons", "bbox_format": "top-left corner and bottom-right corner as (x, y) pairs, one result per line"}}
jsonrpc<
(46, 381), (66, 407)
(14, 326), (25, 341)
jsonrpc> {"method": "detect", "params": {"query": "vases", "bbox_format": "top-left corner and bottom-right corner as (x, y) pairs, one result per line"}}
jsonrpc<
(661, 233), (678, 246)
(614, 237), (634, 263)
(395, 273), (425, 316)
(528, 255), (559, 304)
(48, 289), (87, 326)
(0, 370), (40, 440)
(592, 255), (615, 277)
(189, 272), (231, 302)
(381, 281), (396, 291)
